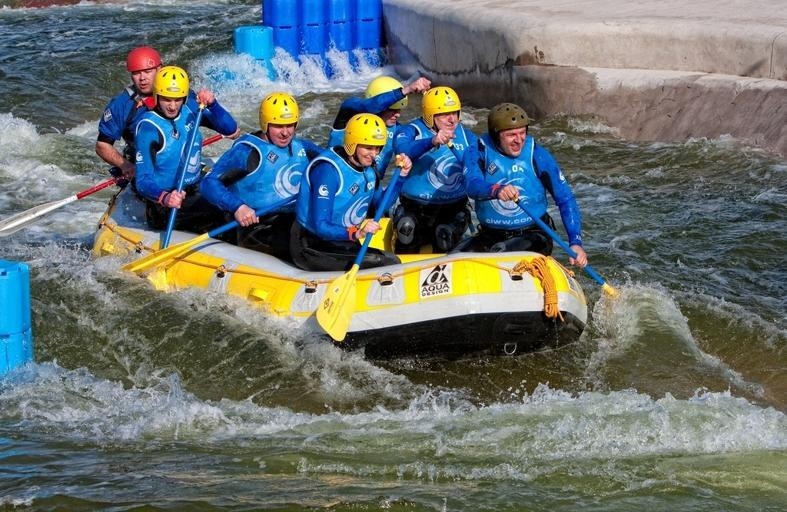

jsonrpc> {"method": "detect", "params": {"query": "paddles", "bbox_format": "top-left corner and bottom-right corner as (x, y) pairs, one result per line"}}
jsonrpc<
(317, 155), (405, 341)
(148, 102), (205, 293)
(1, 134), (228, 233)
(121, 194), (298, 273)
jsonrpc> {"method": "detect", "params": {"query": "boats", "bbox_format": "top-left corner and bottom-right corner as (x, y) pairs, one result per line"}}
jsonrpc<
(90, 202), (589, 359)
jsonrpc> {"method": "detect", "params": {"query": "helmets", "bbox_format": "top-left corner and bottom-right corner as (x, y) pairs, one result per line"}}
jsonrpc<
(154, 66), (189, 99)
(488, 103), (530, 134)
(367, 76), (408, 110)
(345, 114), (387, 157)
(260, 92), (298, 134)
(127, 47), (161, 71)
(422, 87), (461, 128)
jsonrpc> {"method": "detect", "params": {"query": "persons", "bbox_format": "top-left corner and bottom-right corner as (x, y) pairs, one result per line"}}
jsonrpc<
(392, 86), (478, 253)
(288, 112), (413, 271)
(134, 67), (236, 231)
(451, 102), (588, 270)
(328, 76), (433, 178)
(199, 91), (326, 256)
(95, 46), (241, 202)
